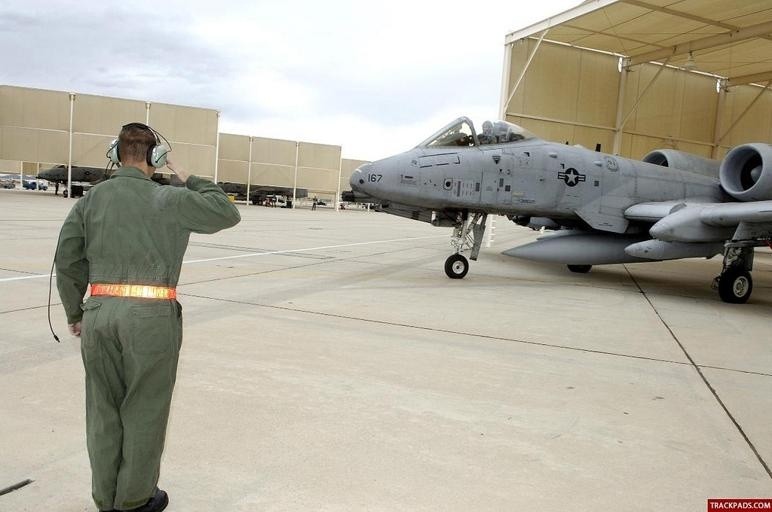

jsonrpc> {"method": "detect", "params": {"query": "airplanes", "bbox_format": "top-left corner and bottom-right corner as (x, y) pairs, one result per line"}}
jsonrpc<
(342, 115), (772, 304)
(36, 164), (116, 195)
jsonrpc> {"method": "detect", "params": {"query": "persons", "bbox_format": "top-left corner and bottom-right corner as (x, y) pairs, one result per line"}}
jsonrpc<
(57, 125), (242, 510)
(271, 193), (277, 207)
(311, 195), (317, 210)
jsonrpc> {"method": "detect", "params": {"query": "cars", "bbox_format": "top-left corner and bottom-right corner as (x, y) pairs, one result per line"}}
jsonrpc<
(24, 181), (49, 191)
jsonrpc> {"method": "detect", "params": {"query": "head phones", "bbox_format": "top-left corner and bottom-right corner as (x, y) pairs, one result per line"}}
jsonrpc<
(106, 122), (173, 169)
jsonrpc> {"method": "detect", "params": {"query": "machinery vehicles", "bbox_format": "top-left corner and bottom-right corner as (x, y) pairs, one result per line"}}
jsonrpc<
(263, 195), (288, 208)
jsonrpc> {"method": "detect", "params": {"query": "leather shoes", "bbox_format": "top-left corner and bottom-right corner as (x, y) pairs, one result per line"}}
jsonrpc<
(114, 490), (169, 512)
